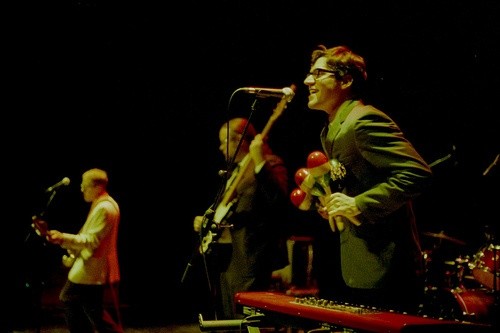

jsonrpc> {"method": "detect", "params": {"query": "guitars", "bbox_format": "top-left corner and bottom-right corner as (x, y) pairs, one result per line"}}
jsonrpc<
(194, 88), (294, 256)
(30, 215), (81, 269)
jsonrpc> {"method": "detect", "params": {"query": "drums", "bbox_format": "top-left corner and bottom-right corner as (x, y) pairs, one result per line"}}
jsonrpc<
(473, 244), (500, 290)
(448, 285), (490, 319)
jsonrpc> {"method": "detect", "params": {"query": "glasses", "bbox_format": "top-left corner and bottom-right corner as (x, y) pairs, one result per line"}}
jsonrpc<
(305, 68), (338, 79)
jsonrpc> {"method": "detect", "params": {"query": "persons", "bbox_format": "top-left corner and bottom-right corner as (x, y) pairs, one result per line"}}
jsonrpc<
(193, 116), (289, 320)
(46, 168), (124, 333)
(303, 46), (422, 317)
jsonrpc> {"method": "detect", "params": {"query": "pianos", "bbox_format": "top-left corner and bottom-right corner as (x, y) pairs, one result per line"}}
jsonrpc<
(236, 286), (487, 333)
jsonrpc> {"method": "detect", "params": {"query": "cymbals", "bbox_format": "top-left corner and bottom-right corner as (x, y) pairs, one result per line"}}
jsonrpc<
(425, 232), (459, 243)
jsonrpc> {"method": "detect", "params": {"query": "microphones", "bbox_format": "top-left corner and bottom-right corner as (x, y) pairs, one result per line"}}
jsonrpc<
(45, 177), (71, 193)
(243, 87), (295, 102)
